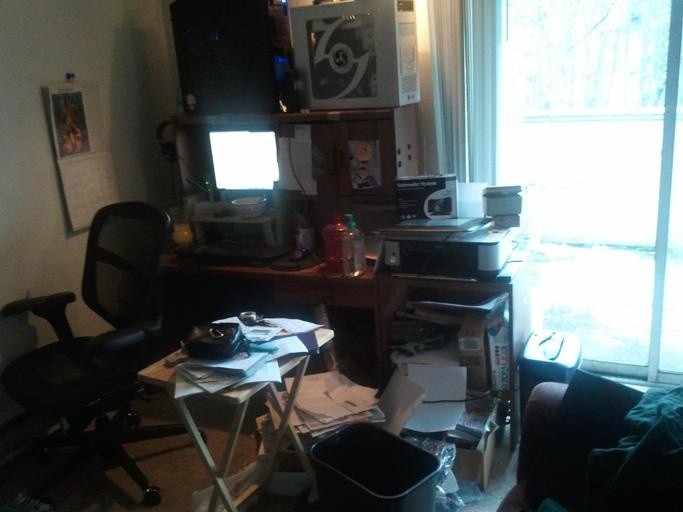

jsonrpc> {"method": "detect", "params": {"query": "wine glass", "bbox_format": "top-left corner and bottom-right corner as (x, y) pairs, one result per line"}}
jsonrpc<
(172, 221), (193, 250)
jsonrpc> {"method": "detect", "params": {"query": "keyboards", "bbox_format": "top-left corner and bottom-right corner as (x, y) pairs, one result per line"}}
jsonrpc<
(197, 244), (287, 265)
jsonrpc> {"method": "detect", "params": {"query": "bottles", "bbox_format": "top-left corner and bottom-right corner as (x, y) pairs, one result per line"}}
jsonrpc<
(271, 56), (294, 113)
(271, 182), (281, 209)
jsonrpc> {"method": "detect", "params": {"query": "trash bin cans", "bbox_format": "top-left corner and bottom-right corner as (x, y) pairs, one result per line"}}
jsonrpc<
(308, 420), (441, 512)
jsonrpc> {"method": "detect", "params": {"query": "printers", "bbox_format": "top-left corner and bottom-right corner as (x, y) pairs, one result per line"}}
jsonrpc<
(383, 219), (513, 280)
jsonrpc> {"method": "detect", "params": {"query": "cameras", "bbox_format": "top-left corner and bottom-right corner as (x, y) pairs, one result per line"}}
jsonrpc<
(238, 310), (267, 327)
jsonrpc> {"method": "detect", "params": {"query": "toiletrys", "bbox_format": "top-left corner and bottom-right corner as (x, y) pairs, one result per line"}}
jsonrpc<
(342, 213), (367, 279)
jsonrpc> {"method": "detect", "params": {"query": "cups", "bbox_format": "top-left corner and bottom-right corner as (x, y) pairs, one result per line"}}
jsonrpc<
(240, 311), (256, 321)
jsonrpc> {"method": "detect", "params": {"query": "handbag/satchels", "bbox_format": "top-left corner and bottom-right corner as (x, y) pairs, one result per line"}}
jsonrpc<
(185, 320), (245, 360)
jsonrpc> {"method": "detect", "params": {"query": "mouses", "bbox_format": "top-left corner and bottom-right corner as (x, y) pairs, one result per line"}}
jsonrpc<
(288, 249), (306, 262)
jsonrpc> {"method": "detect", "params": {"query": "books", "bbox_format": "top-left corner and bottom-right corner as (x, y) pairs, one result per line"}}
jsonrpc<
(183, 350), (269, 378)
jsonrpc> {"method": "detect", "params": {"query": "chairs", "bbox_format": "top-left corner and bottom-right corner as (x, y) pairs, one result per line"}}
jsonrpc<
(1, 201), (171, 507)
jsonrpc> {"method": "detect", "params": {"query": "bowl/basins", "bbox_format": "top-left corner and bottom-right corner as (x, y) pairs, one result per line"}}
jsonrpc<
(231, 197), (268, 218)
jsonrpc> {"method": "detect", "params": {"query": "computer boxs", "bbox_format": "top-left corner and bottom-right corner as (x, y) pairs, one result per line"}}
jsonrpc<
(287, 0), (422, 109)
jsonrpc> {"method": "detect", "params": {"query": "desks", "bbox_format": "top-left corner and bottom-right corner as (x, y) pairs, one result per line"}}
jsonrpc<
(376, 272), (526, 452)
(137, 319), (335, 512)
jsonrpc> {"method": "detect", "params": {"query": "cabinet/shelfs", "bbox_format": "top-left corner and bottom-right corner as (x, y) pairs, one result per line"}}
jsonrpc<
(158, 105), (420, 399)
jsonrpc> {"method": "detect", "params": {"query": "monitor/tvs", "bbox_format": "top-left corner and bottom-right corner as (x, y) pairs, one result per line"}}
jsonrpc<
(205, 124), (280, 204)
